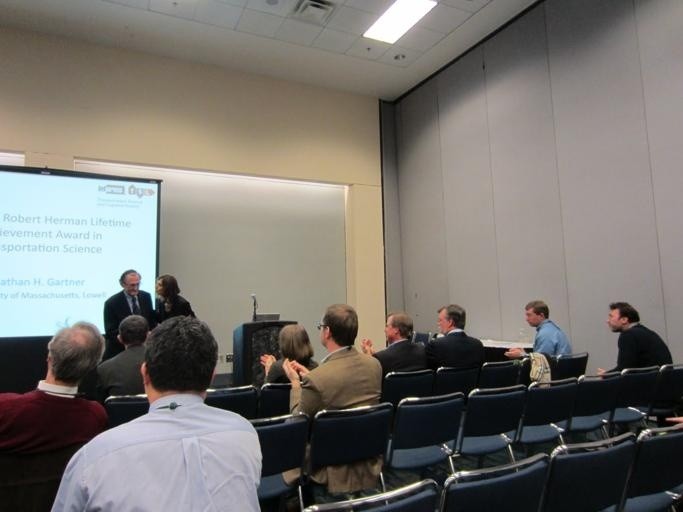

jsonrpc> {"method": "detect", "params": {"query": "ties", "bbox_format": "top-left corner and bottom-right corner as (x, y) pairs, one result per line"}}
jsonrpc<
(132, 297), (140, 315)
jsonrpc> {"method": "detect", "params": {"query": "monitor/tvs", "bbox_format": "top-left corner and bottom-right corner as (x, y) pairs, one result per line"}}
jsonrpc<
(252, 314), (280, 322)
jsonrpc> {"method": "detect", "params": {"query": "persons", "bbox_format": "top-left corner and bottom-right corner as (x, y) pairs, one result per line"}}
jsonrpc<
(427, 303), (487, 368)
(260, 325), (319, 383)
(0, 320), (110, 455)
(154, 274), (197, 326)
(49, 314), (264, 512)
(361, 312), (428, 375)
(105, 268), (155, 357)
(281, 302), (386, 496)
(597, 302), (674, 377)
(503, 299), (572, 361)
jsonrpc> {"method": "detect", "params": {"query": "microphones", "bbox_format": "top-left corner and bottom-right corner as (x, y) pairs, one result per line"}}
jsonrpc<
(250, 293), (257, 321)
